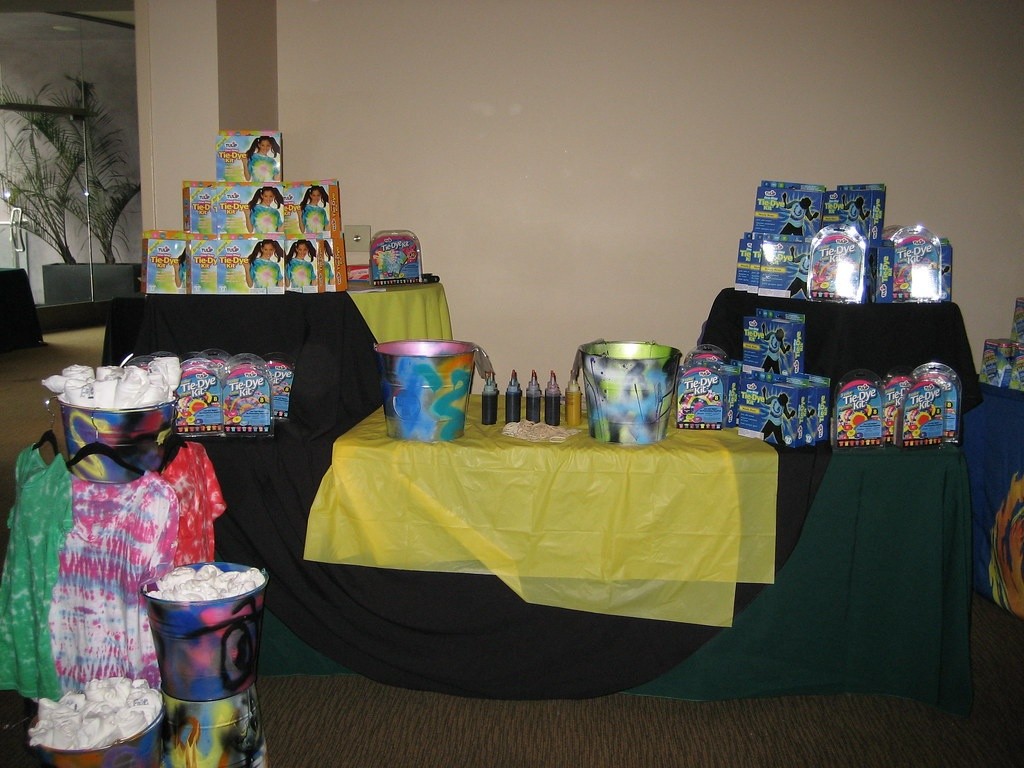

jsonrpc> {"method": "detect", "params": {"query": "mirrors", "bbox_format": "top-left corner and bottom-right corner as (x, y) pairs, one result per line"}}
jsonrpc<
(0, 11), (143, 307)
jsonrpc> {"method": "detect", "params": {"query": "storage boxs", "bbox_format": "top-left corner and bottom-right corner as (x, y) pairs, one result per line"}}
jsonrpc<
(183, 181), (212, 233)
(141, 231), (188, 295)
(186, 233), (217, 294)
(211, 182), (285, 235)
(284, 235), (325, 293)
(216, 235), (285, 294)
(324, 232), (348, 292)
(283, 178), (342, 234)
(215, 131), (283, 182)
(726, 308), (831, 441)
(735, 180), (952, 301)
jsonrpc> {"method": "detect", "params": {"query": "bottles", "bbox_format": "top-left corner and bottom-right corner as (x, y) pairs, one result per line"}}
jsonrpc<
(565, 370), (581, 427)
(505, 368), (523, 424)
(482, 376), (498, 425)
(526, 370), (541, 423)
(545, 370), (561, 426)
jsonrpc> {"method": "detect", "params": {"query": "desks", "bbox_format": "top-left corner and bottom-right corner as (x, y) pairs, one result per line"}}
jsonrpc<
(962, 374), (1024, 622)
(0, 443), (227, 704)
(346, 279), (453, 343)
(214, 446), (972, 719)
(302, 395), (777, 628)
(100, 291), (384, 569)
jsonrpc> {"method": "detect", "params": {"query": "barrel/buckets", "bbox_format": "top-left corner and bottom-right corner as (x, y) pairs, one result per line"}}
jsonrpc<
(579, 341), (683, 445)
(372, 339), (479, 445)
(135, 561), (270, 702)
(57, 394), (180, 485)
(28, 685), (166, 768)
(159, 683), (270, 768)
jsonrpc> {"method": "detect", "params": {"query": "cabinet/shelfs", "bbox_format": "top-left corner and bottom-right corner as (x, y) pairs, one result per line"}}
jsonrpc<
(695, 287), (983, 416)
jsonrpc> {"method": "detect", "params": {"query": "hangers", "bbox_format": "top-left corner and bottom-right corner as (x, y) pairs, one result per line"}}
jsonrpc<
(66, 406), (146, 483)
(32, 396), (60, 456)
(156, 397), (188, 479)
